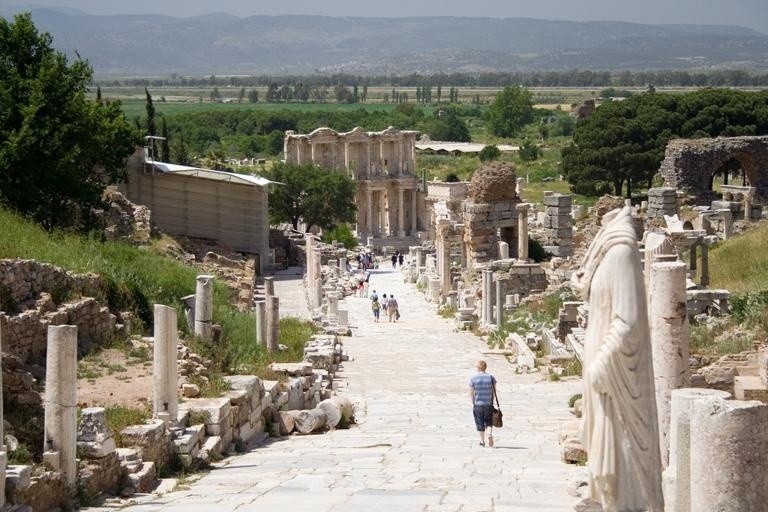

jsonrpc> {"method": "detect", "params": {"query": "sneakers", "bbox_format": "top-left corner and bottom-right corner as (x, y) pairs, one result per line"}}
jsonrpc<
(479, 441), (485, 445)
(489, 436), (493, 446)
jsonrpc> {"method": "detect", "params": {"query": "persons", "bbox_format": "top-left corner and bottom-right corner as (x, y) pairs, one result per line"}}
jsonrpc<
(357, 249), (404, 324)
(468, 359), (497, 447)
(569, 204), (665, 512)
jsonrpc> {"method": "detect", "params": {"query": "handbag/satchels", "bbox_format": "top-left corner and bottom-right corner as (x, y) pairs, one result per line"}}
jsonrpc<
(491, 407), (502, 427)
